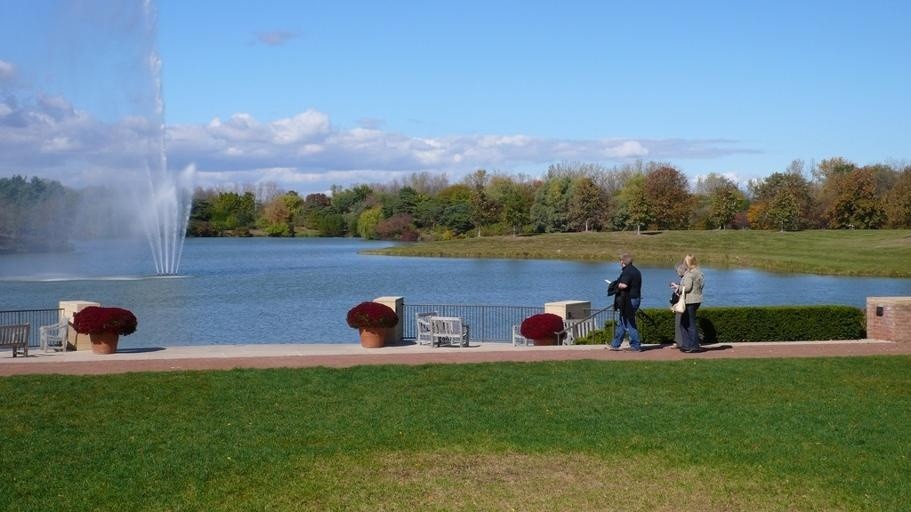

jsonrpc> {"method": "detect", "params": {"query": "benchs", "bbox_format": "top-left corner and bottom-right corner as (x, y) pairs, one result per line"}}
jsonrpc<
(1, 320), (30, 358)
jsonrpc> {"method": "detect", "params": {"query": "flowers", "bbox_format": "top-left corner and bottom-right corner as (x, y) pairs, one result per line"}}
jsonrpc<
(72, 306), (137, 336)
(519, 313), (564, 339)
(345, 302), (398, 329)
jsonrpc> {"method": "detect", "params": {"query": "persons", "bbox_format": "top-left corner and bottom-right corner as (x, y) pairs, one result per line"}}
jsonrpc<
(669, 260), (689, 349)
(669, 254), (705, 353)
(605, 251), (645, 354)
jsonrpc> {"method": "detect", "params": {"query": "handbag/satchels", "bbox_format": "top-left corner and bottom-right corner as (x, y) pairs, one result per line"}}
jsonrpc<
(671, 292), (687, 314)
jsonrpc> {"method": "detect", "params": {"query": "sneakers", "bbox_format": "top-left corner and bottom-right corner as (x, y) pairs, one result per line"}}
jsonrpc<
(625, 346), (641, 352)
(604, 345), (622, 351)
(670, 342), (700, 353)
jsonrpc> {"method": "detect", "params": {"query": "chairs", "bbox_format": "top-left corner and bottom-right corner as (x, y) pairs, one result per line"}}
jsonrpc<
(36, 316), (69, 354)
(414, 312), (469, 348)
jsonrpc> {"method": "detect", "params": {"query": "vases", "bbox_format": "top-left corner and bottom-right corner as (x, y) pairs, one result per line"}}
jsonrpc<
(356, 326), (385, 348)
(88, 331), (119, 354)
(532, 336), (561, 346)
